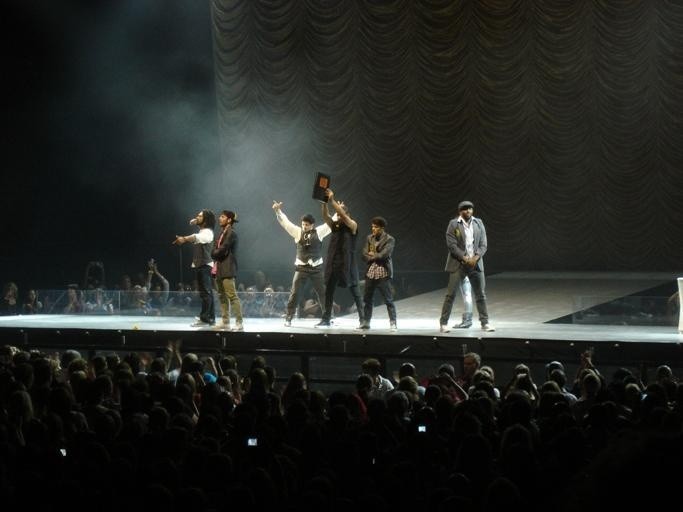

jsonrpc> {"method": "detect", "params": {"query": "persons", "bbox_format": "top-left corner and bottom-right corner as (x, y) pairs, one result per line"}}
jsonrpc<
(314, 188), (364, 326)
(211, 210), (243, 331)
(272, 200), (344, 327)
(356, 216), (397, 331)
(0, 346), (683, 512)
(172, 210), (216, 327)
(439, 201), (495, 333)
(0, 258), (341, 318)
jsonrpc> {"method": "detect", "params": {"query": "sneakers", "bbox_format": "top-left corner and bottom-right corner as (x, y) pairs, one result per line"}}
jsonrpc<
(285, 319), (291, 327)
(315, 320), (330, 328)
(191, 320), (216, 327)
(390, 323), (398, 331)
(481, 324), (495, 331)
(440, 325), (450, 333)
(213, 324), (244, 331)
(356, 325), (370, 331)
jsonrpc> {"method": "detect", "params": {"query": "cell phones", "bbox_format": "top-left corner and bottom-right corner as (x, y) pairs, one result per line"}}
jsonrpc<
(247, 438), (259, 447)
(418, 426), (426, 433)
(60, 449), (67, 456)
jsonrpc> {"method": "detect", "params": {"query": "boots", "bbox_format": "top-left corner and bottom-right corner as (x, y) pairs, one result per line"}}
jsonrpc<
(453, 312), (472, 328)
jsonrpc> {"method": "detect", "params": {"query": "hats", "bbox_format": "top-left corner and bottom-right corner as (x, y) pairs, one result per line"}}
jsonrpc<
(458, 200), (474, 210)
(221, 210), (238, 223)
(301, 213), (315, 222)
(371, 216), (386, 226)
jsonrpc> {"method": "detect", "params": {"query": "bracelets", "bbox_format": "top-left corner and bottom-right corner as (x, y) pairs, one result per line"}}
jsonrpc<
(183, 237), (187, 243)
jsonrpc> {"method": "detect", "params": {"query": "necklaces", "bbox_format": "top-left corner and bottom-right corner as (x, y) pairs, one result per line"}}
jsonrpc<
(304, 231), (313, 240)
